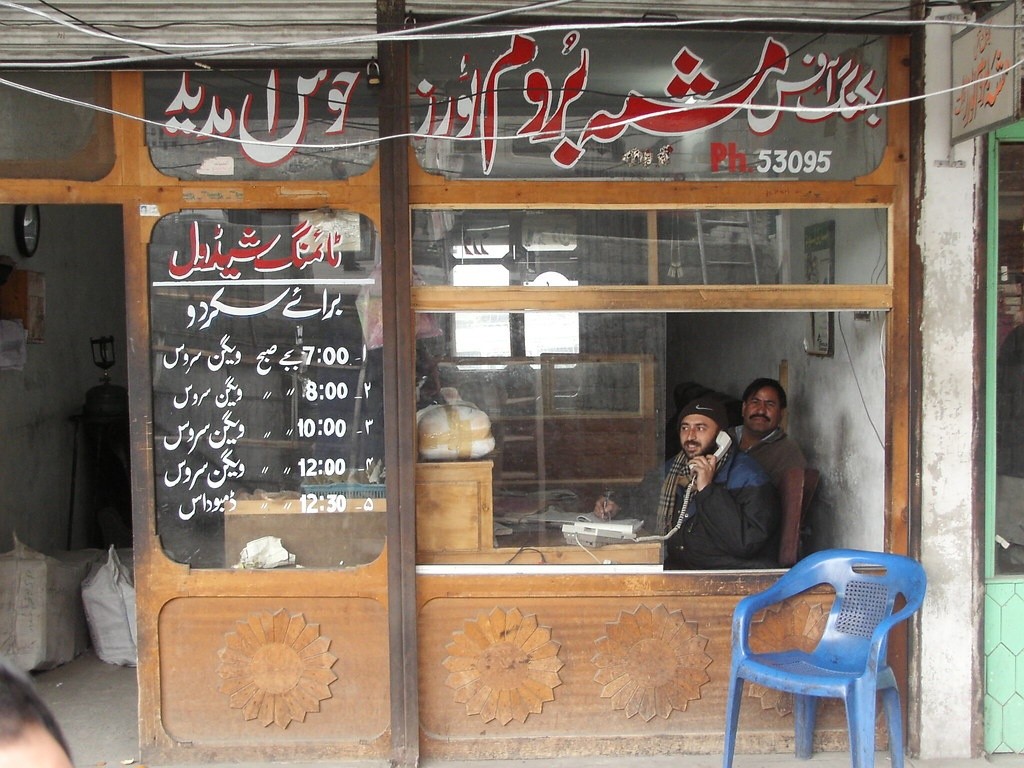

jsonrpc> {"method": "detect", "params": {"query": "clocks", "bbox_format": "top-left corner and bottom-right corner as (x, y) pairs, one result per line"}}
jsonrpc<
(13, 203), (41, 258)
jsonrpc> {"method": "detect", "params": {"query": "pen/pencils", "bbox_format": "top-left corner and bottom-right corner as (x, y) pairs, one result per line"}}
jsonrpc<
(602, 490), (610, 521)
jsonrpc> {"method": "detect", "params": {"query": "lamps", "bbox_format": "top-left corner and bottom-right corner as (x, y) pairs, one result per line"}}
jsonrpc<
(84, 334), (129, 398)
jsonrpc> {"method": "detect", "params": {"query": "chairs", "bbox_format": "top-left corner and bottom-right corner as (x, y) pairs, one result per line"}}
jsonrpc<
(722, 547), (927, 768)
(774, 465), (805, 563)
(801, 469), (821, 526)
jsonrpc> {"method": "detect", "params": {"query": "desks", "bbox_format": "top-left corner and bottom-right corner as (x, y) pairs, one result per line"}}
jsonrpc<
(66, 413), (129, 550)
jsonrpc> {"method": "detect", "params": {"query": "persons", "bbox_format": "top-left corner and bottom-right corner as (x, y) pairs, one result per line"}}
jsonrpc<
(726, 376), (806, 486)
(368, 340), (441, 465)
(594, 396), (783, 570)
(0, 665), (75, 768)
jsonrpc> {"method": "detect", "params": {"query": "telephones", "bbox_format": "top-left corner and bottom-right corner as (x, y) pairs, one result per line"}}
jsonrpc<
(688, 431), (732, 472)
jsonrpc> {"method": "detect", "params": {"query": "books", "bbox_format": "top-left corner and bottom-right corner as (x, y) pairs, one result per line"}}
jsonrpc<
(535, 510), (642, 532)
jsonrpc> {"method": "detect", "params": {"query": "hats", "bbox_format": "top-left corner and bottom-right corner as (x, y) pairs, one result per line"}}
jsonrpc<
(675, 395), (729, 432)
